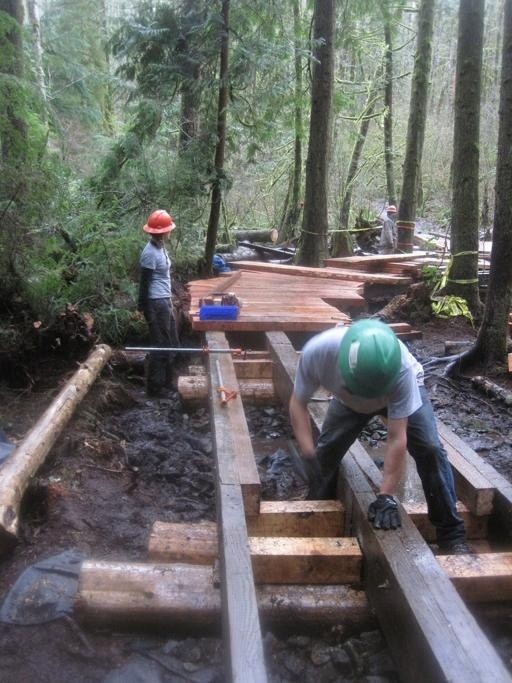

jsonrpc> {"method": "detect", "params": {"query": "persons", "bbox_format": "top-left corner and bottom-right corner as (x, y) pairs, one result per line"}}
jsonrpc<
(380, 205), (399, 254)
(135, 208), (182, 400)
(299, 201), (305, 212)
(211, 253), (230, 272)
(289, 317), (472, 553)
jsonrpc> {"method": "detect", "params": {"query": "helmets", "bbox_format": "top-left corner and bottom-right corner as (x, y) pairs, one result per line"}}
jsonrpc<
(337, 319), (401, 398)
(386, 205), (397, 213)
(143, 210), (176, 234)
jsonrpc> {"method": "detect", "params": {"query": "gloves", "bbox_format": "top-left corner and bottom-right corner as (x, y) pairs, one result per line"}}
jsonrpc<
(301, 452), (324, 483)
(368, 494), (401, 529)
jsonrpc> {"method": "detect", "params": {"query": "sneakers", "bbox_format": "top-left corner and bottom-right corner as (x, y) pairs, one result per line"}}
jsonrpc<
(146, 385), (174, 398)
(439, 542), (477, 553)
